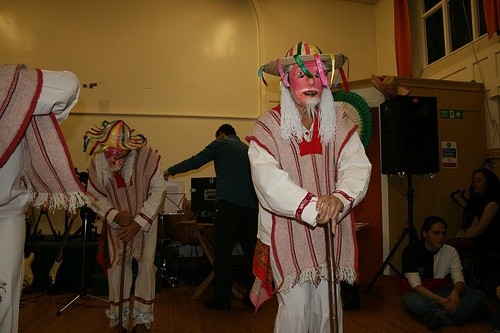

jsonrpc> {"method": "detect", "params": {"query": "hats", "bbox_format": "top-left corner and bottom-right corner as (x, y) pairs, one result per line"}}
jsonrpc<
(83, 119), (147, 160)
(259, 41), (350, 94)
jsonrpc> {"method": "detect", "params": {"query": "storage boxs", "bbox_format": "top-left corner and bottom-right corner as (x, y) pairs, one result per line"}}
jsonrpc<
(190, 176), (216, 222)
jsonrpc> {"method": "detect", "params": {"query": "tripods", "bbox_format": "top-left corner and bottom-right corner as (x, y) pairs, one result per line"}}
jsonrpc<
(55, 189), (110, 316)
(367, 175), (417, 293)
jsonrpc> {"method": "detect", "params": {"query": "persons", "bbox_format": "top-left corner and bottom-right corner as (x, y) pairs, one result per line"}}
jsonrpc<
(0, 64), (81, 333)
(245, 42), (373, 333)
(163, 123), (260, 311)
(402, 168), (500, 333)
(84, 120), (167, 333)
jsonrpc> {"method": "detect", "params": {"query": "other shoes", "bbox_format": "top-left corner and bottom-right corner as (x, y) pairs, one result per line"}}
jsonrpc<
(245, 297), (254, 308)
(204, 298), (231, 311)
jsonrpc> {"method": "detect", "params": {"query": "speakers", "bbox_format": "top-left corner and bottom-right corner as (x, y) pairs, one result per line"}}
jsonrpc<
(380, 95), (440, 174)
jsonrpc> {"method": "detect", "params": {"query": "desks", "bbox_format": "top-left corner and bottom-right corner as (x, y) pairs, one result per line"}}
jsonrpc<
(177, 220), (245, 301)
